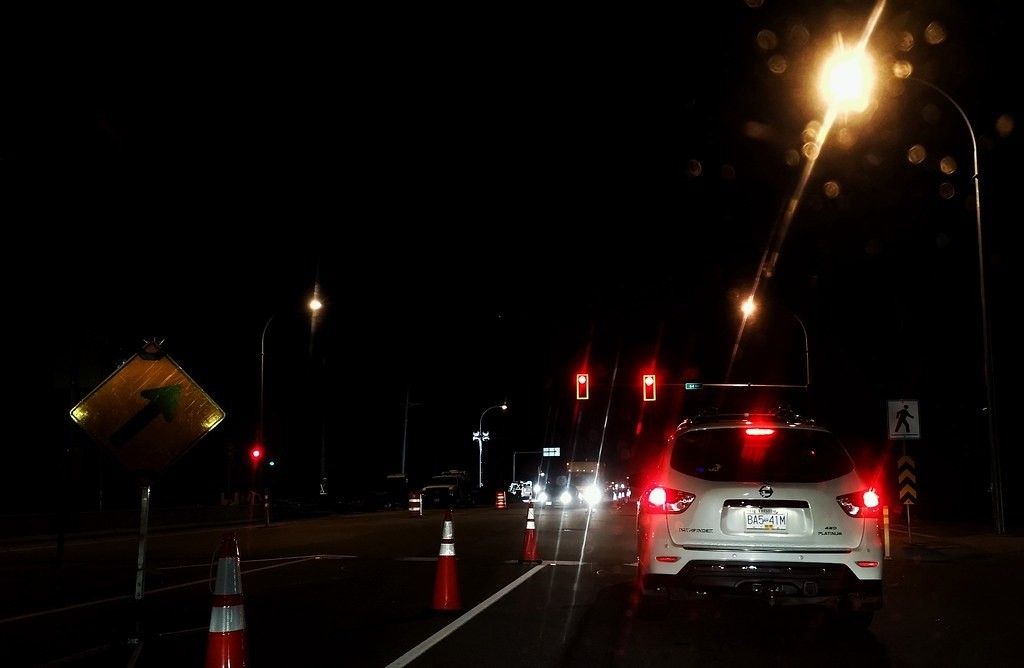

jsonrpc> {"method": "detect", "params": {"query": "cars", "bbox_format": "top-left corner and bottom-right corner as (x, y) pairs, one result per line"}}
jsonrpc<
(535, 472), (631, 510)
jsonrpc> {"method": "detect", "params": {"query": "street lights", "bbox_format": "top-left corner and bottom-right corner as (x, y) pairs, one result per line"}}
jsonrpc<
(254, 295), (324, 496)
(740, 297), (811, 388)
(478, 402), (507, 489)
(816, 49), (1007, 536)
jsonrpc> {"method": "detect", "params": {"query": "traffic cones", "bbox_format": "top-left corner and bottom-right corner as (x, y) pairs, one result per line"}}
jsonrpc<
(423, 504), (471, 616)
(518, 500), (543, 565)
(203, 527), (251, 668)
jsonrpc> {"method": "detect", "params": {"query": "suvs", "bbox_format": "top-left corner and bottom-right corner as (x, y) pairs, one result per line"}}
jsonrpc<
(626, 405), (886, 630)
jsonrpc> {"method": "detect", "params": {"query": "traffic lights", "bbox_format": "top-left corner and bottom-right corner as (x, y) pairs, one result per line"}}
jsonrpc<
(643, 374), (657, 402)
(577, 373), (589, 401)
